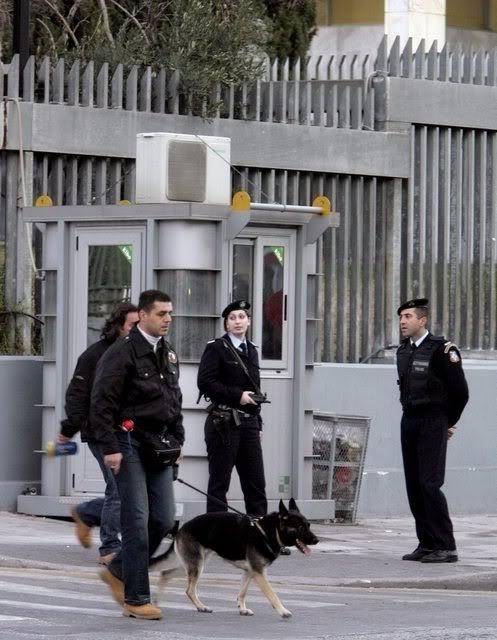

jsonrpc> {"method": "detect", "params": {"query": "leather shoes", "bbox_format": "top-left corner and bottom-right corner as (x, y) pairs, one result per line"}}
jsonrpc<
(419, 546), (458, 563)
(122, 601), (164, 620)
(101, 570), (127, 607)
(403, 544), (436, 561)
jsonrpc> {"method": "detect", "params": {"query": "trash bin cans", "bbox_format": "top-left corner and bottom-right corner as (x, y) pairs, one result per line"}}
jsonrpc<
(309, 412), (372, 525)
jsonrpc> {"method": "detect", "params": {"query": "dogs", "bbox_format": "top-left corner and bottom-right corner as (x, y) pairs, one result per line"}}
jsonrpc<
(149, 497), (320, 619)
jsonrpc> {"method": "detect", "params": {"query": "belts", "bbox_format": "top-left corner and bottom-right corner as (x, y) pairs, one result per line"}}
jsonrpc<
(237, 412), (255, 419)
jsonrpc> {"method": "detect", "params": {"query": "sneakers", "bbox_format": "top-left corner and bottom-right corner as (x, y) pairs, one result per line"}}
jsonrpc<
(70, 505), (92, 549)
(98, 551), (119, 566)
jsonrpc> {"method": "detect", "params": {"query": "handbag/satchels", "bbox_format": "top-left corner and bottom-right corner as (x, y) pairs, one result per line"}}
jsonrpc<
(130, 429), (181, 470)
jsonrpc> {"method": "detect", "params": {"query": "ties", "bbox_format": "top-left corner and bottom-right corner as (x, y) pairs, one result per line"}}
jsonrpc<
(408, 342), (418, 359)
(239, 343), (248, 359)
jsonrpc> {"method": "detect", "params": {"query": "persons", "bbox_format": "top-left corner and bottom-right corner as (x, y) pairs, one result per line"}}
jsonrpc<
(85, 287), (186, 625)
(53, 301), (141, 569)
(195, 300), (272, 517)
(393, 294), (472, 565)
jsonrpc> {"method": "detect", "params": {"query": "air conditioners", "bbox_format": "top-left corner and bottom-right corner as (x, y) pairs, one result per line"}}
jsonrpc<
(132, 128), (234, 204)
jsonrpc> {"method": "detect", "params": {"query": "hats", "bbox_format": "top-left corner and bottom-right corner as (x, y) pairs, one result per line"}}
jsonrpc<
(221, 300), (251, 318)
(397, 297), (430, 318)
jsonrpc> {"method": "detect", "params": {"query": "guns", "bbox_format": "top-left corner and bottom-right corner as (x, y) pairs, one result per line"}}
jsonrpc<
(196, 387), (271, 426)
